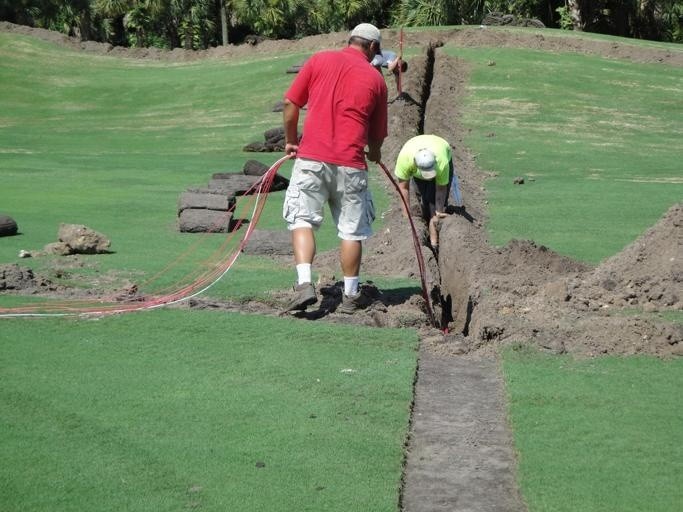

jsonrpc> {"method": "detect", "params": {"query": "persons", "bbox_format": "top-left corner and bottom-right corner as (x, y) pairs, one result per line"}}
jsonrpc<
(370, 50), (407, 92)
(394, 134), (453, 217)
(277, 23), (387, 317)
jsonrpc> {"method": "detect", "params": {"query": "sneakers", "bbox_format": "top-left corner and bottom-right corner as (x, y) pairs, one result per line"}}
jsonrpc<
(278, 282), (317, 315)
(341, 288), (372, 314)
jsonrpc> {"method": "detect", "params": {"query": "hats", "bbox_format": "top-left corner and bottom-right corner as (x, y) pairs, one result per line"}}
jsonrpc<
(414, 149), (436, 180)
(351, 23), (384, 57)
